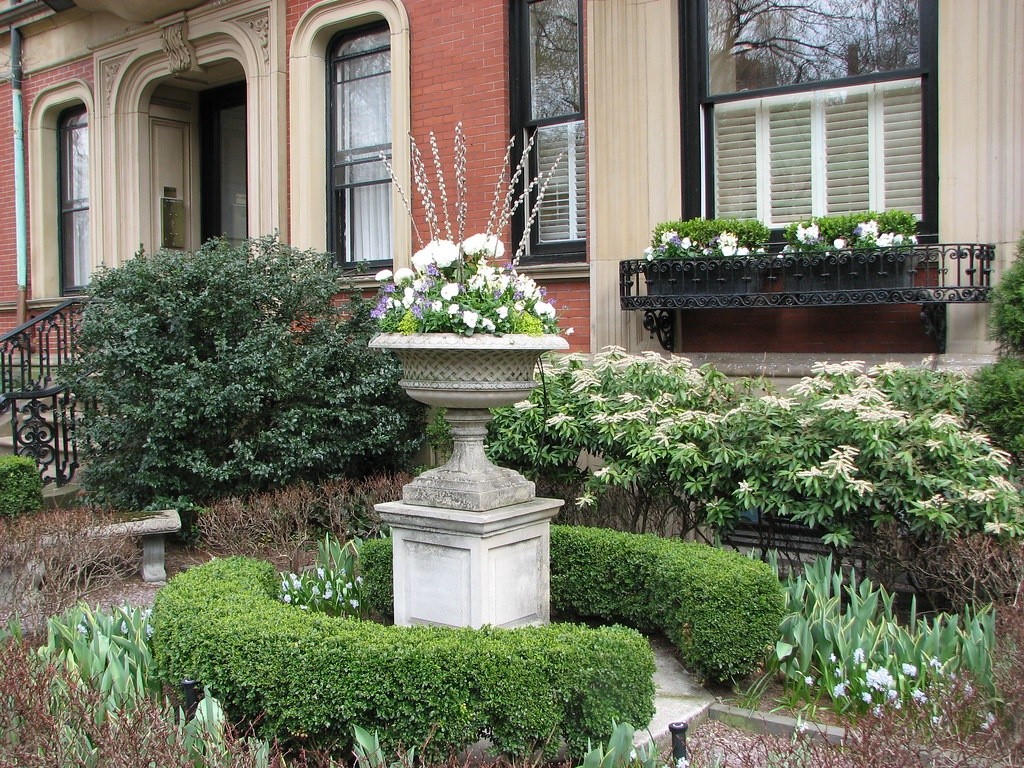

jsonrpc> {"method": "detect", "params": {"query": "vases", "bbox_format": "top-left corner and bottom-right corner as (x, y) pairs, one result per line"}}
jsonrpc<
(782, 252), (918, 293)
(367, 330), (569, 511)
(641, 257), (767, 296)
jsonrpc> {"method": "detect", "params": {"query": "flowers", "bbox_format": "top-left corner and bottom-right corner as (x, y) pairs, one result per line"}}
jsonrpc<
(778, 211), (920, 255)
(642, 217), (770, 262)
(369, 124), (575, 338)
